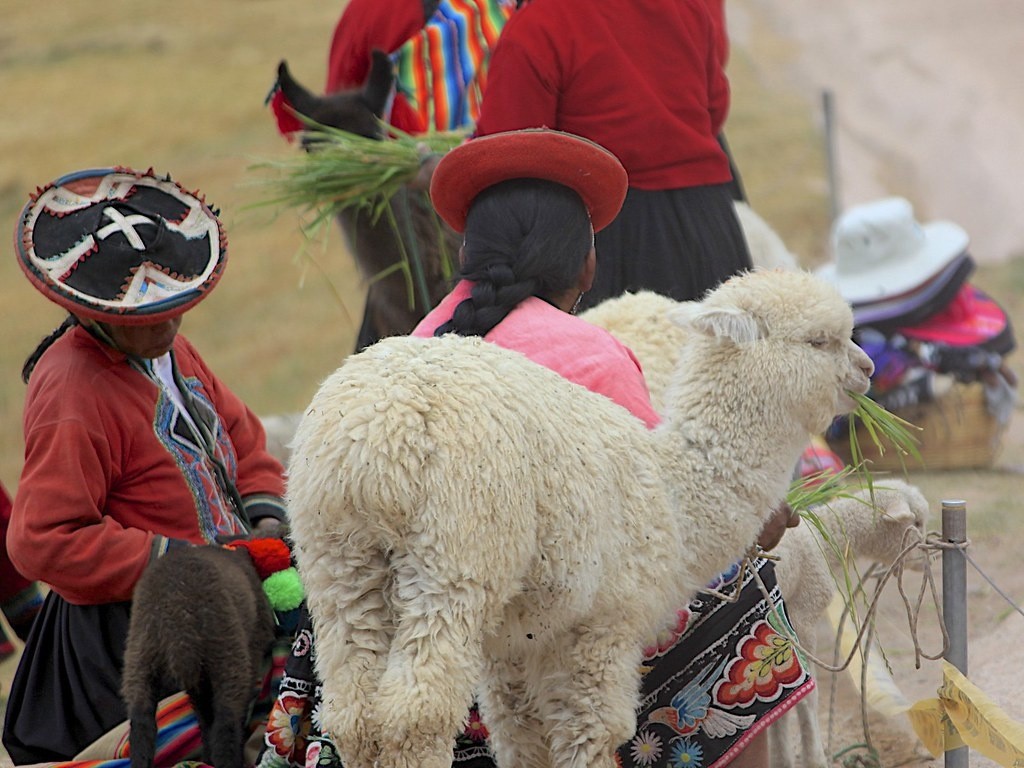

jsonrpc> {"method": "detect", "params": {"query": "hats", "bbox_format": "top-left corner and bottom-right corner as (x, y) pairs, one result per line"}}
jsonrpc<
(812, 198), (973, 332)
(11, 165), (229, 327)
(429, 126), (629, 234)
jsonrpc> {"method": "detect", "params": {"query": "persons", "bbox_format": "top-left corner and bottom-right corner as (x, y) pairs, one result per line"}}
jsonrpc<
(259, 128), (931, 768)
(0, 167), (286, 767)
(266, 0), (754, 358)
